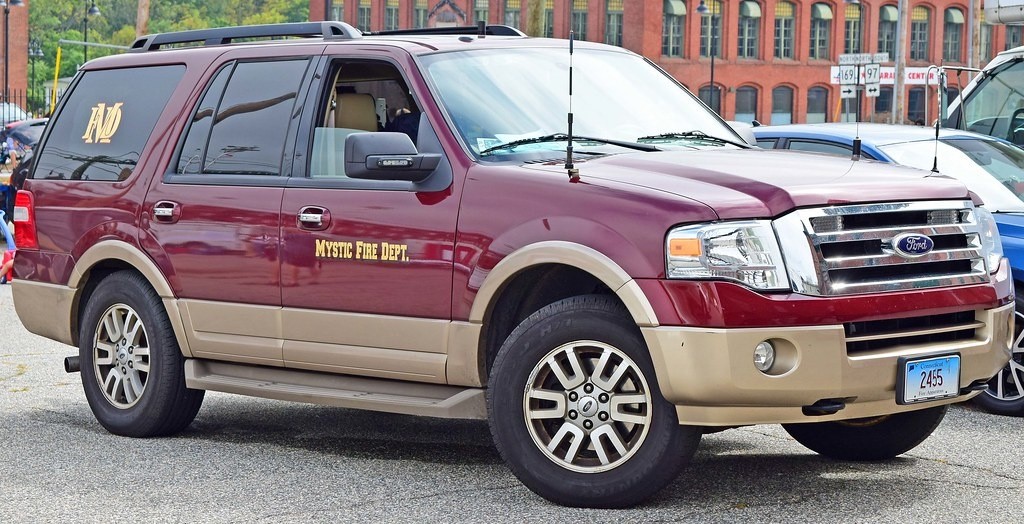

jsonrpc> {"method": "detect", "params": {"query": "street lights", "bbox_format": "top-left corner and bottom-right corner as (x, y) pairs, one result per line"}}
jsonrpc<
(28, 38), (45, 118)
(85, 0), (100, 66)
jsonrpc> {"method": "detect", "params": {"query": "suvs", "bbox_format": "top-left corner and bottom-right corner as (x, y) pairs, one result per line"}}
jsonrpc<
(13, 19), (1015, 513)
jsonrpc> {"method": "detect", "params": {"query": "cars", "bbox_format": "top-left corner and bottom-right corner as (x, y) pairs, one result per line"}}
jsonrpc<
(743, 119), (1024, 417)
(0, 103), (30, 128)
(935, 46), (1024, 153)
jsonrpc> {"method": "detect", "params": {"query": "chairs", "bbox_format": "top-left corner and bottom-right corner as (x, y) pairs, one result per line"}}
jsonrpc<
(334, 93), (377, 174)
